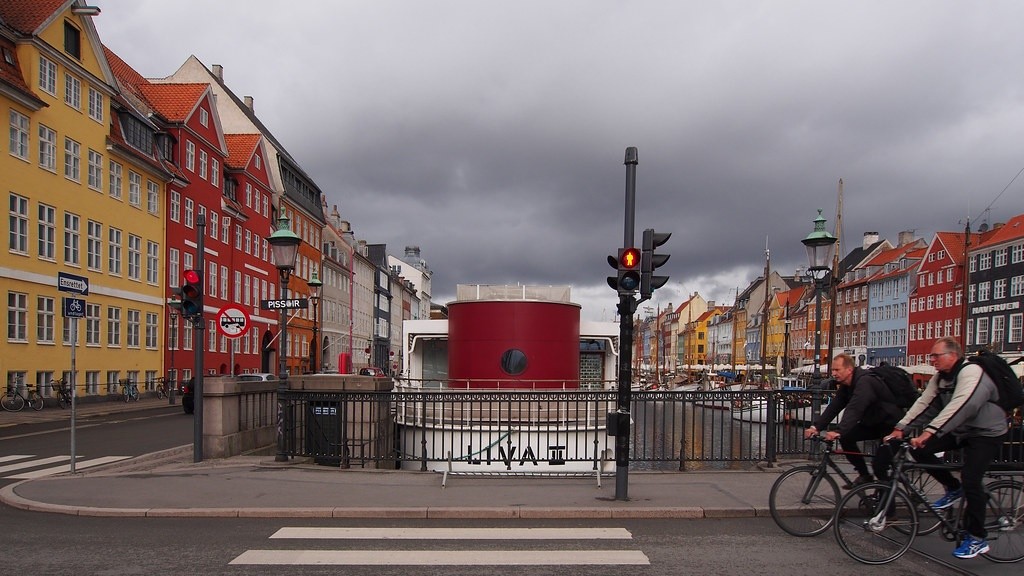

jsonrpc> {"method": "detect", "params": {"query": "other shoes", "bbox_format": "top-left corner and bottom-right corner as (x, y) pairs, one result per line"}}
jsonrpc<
(842, 475), (874, 490)
(864, 508), (897, 523)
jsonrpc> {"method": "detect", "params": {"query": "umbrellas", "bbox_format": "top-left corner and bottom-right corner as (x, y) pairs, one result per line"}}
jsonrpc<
(791, 363), (937, 389)
(678, 363), (784, 376)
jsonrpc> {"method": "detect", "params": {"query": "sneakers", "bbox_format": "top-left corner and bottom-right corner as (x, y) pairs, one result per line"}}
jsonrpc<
(952, 536), (990, 558)
(931, 485), (965, 509)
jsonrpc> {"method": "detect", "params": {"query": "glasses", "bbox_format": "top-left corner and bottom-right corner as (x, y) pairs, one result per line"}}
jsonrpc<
(929, 351), (953, 361)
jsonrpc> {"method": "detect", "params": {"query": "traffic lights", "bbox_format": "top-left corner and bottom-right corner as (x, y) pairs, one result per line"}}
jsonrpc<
(167, 288), (190, 318)
(617, 248), (642, 292)
(642, 228), (672, 300)
(607, 255), (618, 289)
(181, 270), (202, 316)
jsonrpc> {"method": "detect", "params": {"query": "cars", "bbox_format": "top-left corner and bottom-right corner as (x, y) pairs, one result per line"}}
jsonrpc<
(360, 367), (385, 377)
(182, 375), (245, 414)
(237, 373), (279, 381)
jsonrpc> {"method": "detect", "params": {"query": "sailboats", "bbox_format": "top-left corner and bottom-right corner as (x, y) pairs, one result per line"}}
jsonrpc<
(631, 178), (846, 430)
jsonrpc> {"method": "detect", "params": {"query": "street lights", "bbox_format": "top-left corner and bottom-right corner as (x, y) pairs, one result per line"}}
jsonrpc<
(801, 207), (839, 461)
(268, 207), (302, 461)
(306, 266), (324, 374)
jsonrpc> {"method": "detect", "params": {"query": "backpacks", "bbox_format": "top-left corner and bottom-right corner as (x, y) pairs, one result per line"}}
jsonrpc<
(853, 360), (921, 408)
(937, 349), (1024, 413)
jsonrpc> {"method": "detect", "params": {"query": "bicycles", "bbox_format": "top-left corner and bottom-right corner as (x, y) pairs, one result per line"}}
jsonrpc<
(768, 432), (954, 537)
(153, 377), (170, 400)
(0, 375), (45, 412)
(833, 436), (1024, 563)
(50, 378), (72, 410)
(119, 378), (140, 403)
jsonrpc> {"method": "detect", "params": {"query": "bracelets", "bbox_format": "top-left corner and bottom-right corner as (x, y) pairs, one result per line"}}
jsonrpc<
(810, 425), (817, 431)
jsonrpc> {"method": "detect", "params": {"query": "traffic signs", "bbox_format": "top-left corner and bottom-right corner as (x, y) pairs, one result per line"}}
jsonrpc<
(57, 271), (89, 297)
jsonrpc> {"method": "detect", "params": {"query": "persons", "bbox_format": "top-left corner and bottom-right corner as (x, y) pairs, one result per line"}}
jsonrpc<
(881, 336), (1009, 559)
(805, 352), (905, 522)
